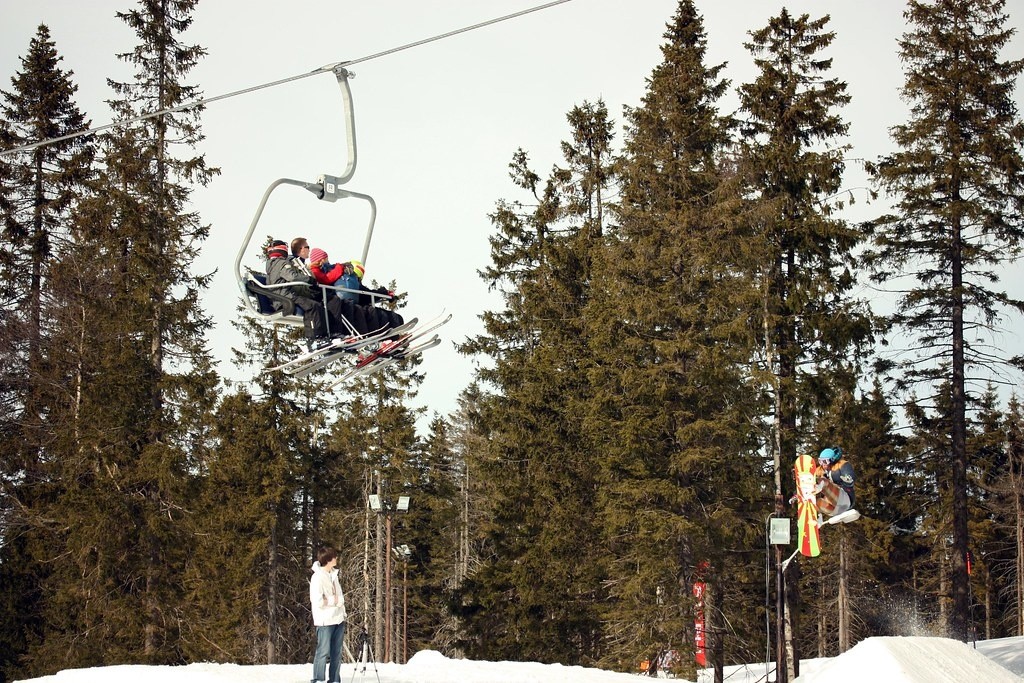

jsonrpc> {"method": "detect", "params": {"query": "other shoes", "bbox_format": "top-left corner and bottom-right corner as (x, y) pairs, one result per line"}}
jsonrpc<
(316, 336), (332, 350)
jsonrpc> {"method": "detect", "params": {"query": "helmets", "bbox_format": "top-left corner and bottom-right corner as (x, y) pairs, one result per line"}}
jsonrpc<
(350, 260), (364, 278)
(268, 240), (288, 258)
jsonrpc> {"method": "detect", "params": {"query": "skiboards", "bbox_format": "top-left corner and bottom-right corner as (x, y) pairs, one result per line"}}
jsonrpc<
(261, 306), (459, 402)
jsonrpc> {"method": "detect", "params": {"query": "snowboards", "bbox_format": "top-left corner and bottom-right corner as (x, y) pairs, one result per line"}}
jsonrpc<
(794, 454), (823, 558)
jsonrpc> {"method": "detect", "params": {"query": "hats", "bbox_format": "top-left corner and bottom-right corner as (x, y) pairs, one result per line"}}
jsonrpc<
(818, 449), (834, 459)
(310, 248), (328, 263)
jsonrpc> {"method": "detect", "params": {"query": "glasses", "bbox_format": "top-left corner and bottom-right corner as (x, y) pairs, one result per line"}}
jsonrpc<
(818, 459), (832, 466)
(300, 246), (309, 249)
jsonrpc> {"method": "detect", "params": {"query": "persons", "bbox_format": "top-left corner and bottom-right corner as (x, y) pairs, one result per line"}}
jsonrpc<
(309, 546), (347, 683)
(266, 237), (410, 359)
(788, 448), (855, 530)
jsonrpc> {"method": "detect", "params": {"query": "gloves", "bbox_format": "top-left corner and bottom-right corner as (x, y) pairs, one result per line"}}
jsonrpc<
(308, 275), (319, 290)
(388, 290), (395, 300)
